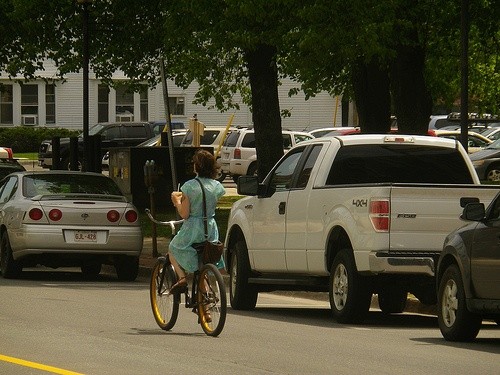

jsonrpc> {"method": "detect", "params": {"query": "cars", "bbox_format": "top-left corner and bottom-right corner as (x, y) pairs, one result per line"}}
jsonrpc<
(0, 171), (143, 283)
(434, 190), (500, 342)
(429, 113), (500, 184)
(310, 126), (361, 139)
(0, 147), (27, 187)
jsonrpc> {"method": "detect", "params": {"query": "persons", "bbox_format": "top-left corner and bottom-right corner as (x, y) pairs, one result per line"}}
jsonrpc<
(168, 150), (226, 322)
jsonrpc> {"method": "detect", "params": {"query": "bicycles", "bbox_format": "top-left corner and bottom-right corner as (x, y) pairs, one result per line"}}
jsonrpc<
(144, 208), (227, 336)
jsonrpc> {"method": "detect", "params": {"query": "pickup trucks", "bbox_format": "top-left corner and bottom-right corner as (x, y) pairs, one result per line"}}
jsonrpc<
(221, 133), (500, 325)
(37, 121), (186, 172)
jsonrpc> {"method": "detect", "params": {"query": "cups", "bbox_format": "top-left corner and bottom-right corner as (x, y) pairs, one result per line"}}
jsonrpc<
(173, 191), (182, 207)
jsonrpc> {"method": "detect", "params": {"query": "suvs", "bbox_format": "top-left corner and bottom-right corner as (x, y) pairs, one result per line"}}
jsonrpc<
(102, 125), (238, 184)
(220, 126), (317, 184)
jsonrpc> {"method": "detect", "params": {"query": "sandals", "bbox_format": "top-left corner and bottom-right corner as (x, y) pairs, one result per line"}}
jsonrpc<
(198, 314), (212, 323)
(169, 277), (188, 294)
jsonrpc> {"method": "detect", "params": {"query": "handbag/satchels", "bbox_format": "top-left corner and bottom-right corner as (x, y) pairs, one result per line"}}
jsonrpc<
(191, 240), (224, 268)
